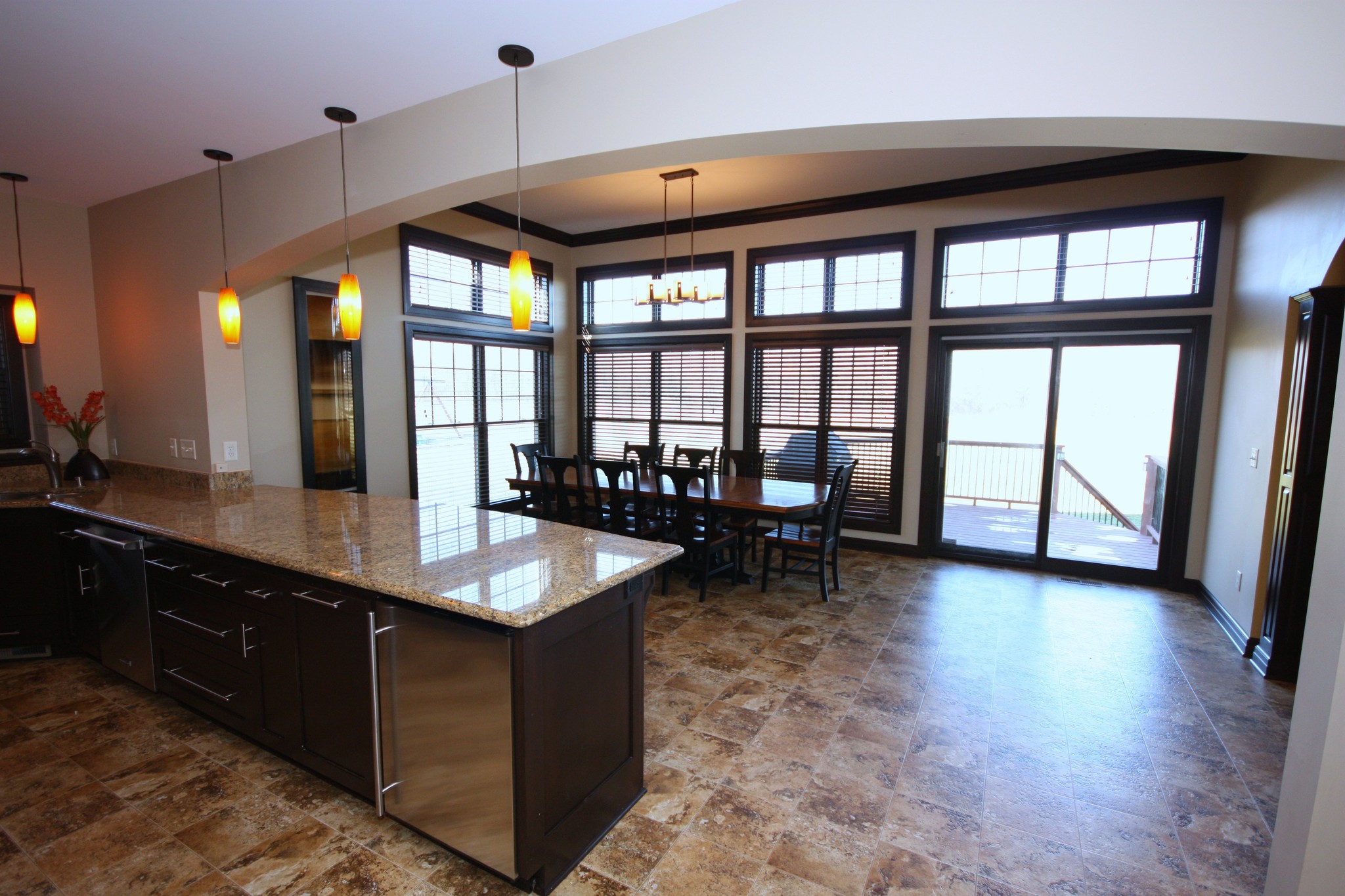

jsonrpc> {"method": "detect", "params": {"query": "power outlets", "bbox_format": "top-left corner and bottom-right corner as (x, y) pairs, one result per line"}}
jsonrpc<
(223, 441), (239, 462)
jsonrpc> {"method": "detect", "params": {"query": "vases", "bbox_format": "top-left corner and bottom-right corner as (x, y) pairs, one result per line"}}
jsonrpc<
(62, 448), (110, 480)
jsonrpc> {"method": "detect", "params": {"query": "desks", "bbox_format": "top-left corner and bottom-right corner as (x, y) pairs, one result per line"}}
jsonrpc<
(505, 465), (838, 585)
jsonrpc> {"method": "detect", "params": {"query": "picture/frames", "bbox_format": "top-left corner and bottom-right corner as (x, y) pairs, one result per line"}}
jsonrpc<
(292, 276), (368, 495)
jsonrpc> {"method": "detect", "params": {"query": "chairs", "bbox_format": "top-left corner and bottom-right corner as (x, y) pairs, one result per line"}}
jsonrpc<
(509, 436), (588, 523)
(534, 440), (767, 602)
(761, 458), (859, 601)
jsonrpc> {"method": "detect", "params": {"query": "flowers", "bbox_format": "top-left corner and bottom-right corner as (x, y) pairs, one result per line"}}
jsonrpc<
(32, 384), (107, 449)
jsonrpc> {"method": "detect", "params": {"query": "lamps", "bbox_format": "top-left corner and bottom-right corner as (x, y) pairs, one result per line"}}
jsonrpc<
(497, 44), (535, 332)
(0, 171), (38, 345)
(203, 148), (241, 343)
(635, 168), (727, 305)
(324, 106), (363, 341)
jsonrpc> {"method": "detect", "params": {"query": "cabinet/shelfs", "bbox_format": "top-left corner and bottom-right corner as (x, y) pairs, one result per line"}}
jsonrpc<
(0, 507), (76, 659)
(143, 539), (647, 896)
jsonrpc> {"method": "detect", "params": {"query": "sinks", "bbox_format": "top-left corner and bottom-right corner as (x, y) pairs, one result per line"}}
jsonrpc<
(0, 485), (97, 503)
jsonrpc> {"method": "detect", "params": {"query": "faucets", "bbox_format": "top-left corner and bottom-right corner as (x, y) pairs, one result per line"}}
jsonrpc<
(17, 439), (63, 488)
